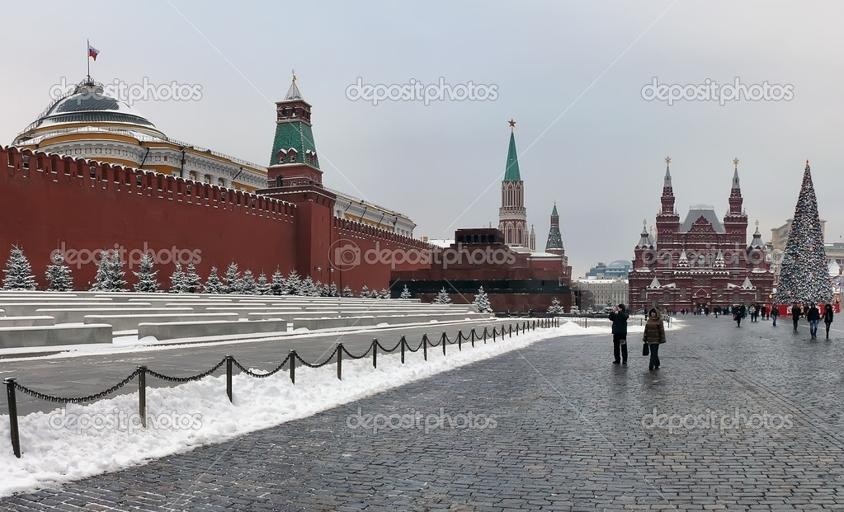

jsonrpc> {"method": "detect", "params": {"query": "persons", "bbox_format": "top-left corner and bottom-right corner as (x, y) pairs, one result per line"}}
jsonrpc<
(666, 301), (810, 335)
(821, 303), (833, 339)
(807, 302), (820, 339)
(642, 307), (666, 370)
(608, 303), (629, 366)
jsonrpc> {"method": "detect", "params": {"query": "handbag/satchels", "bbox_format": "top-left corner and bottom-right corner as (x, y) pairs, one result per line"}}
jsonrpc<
(643, 343), (649, 355)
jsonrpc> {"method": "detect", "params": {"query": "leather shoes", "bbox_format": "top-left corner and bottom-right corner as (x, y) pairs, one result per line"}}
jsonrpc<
(613, 360), (627, 364)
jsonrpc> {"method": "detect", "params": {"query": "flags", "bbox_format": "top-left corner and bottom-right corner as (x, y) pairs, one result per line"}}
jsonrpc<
(90, 45), (100, 62)
